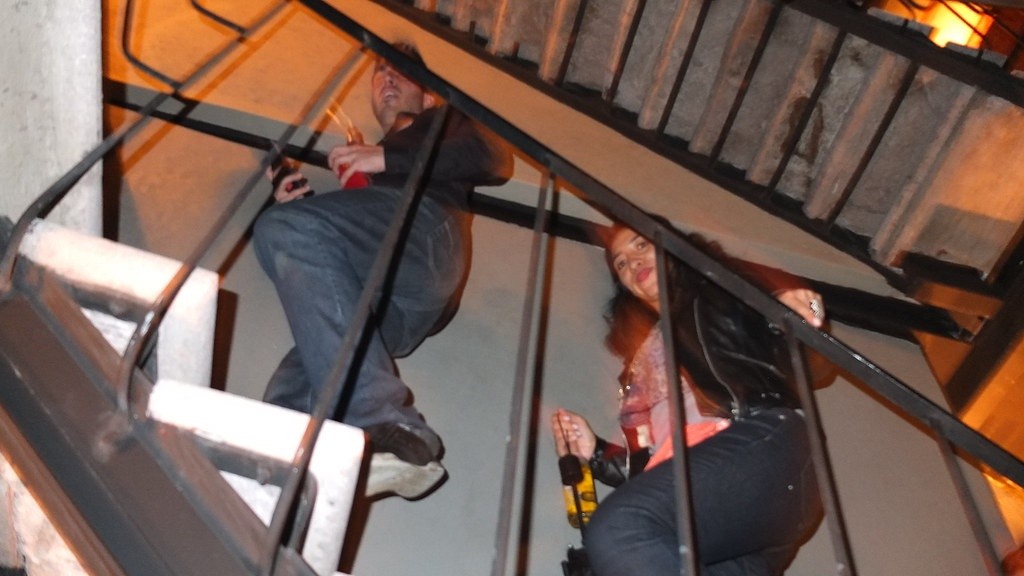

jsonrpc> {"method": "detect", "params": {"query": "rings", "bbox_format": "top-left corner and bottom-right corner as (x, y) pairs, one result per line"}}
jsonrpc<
(808, 300), (823, 319)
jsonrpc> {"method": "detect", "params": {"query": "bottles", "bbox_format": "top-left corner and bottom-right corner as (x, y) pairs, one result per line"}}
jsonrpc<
(339, 129), (369, 191)
(269, 152), (306, 205)
(559, 439), (598, 529)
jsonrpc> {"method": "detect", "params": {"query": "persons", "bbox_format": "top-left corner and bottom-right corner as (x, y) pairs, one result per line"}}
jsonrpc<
(249, 42), (519, 503)
(547, 211), (827, 576)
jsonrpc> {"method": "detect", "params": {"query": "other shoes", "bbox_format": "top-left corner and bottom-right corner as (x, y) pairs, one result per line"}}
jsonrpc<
(361, 425), (447, 501)
